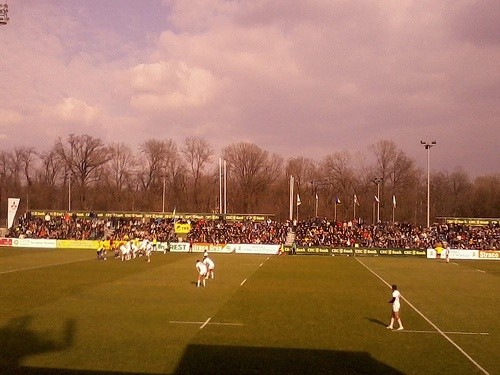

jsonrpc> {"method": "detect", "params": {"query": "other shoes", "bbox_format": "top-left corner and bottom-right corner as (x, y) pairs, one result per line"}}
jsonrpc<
(205, 277), (208, 279)
(396, 327), (404, 330)
(386, 326), (393, 330)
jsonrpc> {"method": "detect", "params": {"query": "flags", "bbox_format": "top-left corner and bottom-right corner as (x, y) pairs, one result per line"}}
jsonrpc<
(316, 195), (319, 204)
(354, 194), (360, 206)
(374, 195), (380, 203)
(393, 194), (397, 208)
(336, 194), (341, 204)
(296, 193), (301, 205)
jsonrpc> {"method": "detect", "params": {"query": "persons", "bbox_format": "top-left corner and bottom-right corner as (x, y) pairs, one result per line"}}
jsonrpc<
(5, 213), (500, 287)
(387, 285), (404, 330)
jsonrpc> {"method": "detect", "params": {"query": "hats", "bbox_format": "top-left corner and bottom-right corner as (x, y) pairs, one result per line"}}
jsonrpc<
(204, 252), (208, 256)
